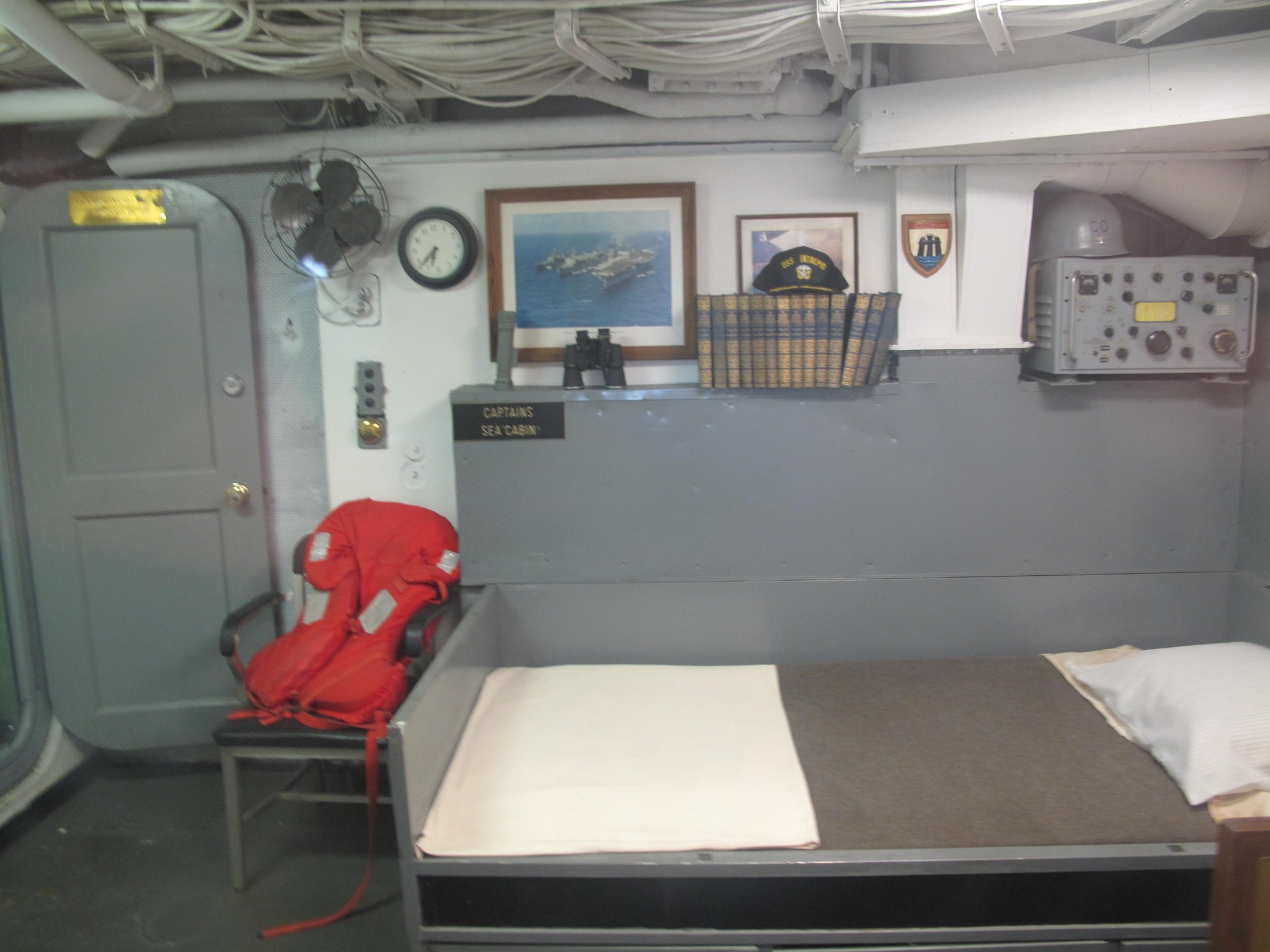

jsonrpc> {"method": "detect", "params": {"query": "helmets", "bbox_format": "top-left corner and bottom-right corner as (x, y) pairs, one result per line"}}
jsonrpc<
(1030, 191), (1130, 262)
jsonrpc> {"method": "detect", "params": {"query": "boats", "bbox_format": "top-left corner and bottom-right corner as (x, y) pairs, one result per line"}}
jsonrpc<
(536, 239), (656, 288)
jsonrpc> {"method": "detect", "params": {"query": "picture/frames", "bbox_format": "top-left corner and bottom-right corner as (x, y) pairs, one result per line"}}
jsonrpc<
(736, 211), (858, 296)
(484, 181), (700, 362)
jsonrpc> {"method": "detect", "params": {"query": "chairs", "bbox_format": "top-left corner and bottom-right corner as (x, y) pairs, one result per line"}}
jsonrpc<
(209, 532), (460, 893)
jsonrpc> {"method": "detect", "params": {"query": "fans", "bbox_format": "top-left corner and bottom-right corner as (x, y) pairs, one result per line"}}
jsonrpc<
(260, 145), (392, 281)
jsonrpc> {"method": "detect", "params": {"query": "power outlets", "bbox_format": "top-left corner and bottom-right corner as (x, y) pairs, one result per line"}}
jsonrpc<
(349, 274), (382, 326)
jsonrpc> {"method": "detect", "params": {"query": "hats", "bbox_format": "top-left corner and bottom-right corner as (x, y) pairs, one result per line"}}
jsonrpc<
(754, 246), (849, 292)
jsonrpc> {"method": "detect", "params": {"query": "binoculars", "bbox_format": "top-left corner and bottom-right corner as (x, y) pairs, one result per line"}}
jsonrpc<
(562, 328), (628, 390)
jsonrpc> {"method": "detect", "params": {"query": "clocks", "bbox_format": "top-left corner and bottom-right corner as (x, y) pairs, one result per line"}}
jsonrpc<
(397, 206), (480, 289)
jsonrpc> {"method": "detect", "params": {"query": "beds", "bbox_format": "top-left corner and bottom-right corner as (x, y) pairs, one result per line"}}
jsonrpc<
(388, 587), (1270, 951)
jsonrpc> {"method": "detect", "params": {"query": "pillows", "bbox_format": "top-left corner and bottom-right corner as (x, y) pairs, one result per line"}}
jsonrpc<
(1066, 643), (1270, 805)
